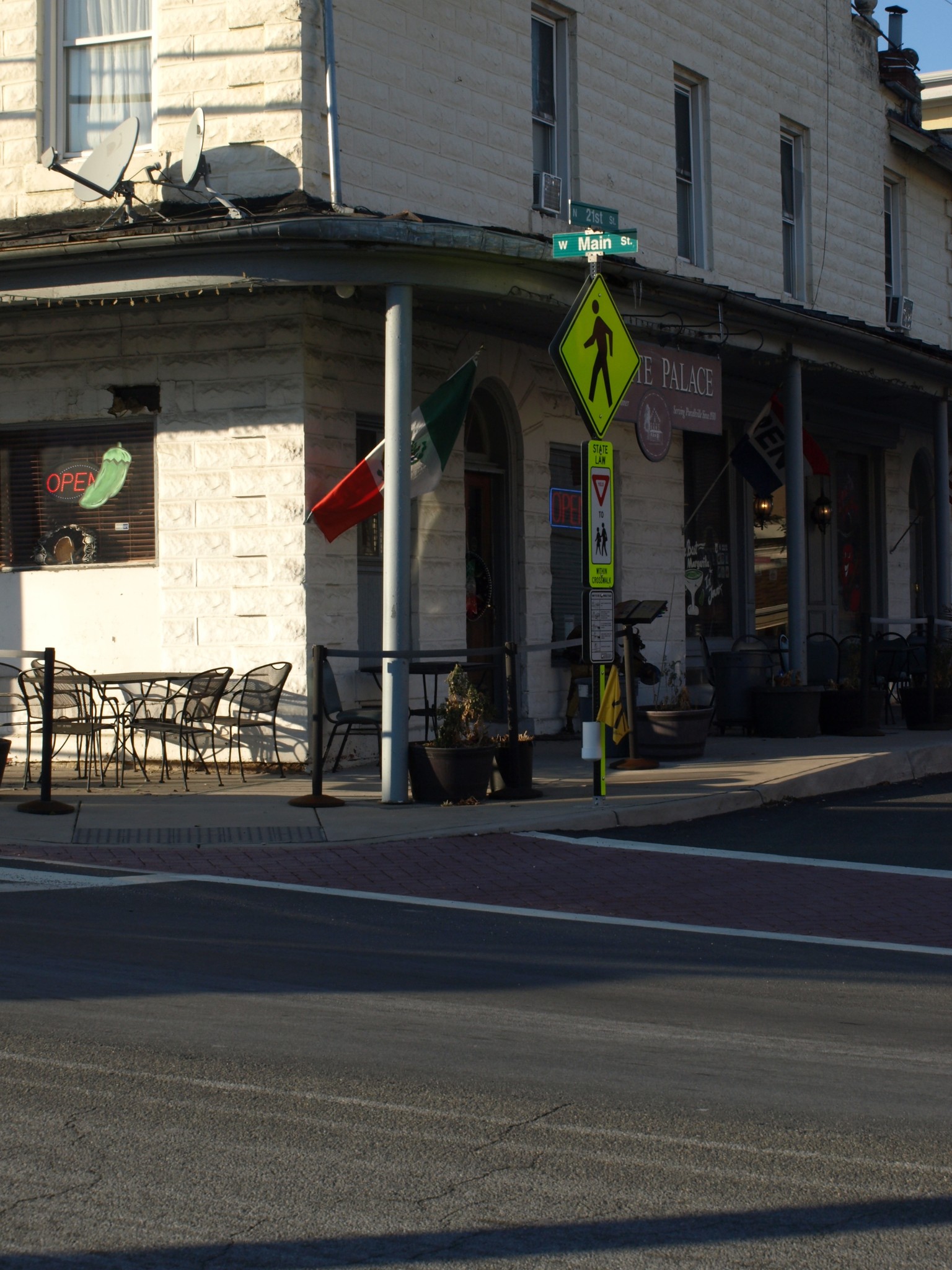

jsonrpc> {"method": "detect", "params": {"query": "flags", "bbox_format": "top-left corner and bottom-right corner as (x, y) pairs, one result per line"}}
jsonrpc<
(730, 394), (830, 495)
(310, 355), (481, 544)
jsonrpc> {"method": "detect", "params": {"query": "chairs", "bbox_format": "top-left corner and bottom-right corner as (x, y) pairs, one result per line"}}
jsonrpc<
(311, 644), (383, 781)
(0, 658), (292, 791)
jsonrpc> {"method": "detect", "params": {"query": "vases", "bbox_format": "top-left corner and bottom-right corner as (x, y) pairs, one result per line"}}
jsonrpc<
(492, 741), (536, 798)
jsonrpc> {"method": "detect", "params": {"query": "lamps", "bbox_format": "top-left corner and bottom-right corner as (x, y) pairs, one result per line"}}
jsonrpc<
(621, 311), (684, 348)
(810, 486), (832, 536)
(698, 328), (764, 360)
(659, 321), (729, 357)
(753, 490), (774, 531)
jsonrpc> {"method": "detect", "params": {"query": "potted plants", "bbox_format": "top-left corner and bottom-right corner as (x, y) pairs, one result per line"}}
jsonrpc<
(825, 644), (952, 737)
(758, 668), (825, 740)
(634, 650), (712, 762)
(406, 662), (499, 806)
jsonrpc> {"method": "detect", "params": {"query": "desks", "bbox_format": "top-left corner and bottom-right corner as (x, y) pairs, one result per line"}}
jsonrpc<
(22, 671), (224, 788)
(357, 661), (489, 739)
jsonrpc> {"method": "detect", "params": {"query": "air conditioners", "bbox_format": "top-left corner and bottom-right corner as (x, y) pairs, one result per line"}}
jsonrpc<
(532, 172), (562, 216)
(885, 294), (914, 331)
